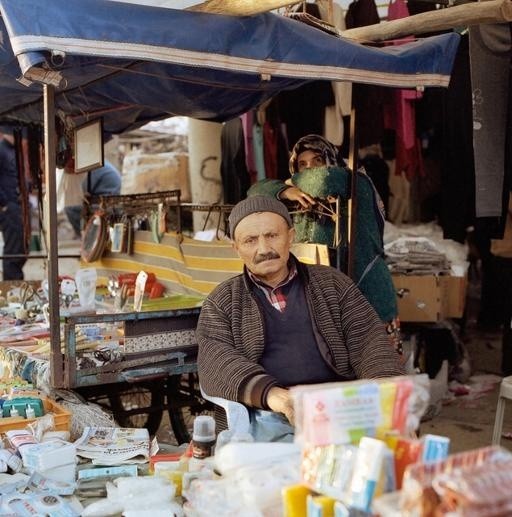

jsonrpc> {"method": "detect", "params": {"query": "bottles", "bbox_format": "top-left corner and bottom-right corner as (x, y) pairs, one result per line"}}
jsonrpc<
(192, 415), (216, 460)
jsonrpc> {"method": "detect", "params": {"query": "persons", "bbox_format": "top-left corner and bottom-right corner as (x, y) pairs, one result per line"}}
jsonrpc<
(246, 133), (405, 365)
(63, 157), (122, 239)
(194, 194), (409, 443)
(356, 144), (389, 218)
(1, 130), (35, 283)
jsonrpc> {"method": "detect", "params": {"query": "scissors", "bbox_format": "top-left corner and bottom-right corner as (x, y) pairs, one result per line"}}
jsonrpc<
(61, 293), (73, 308)
(19, 282), (34, 305)
(121, 282), (130, 313)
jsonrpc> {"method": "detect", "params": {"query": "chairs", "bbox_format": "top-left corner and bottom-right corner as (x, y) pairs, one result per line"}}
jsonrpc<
(198, 379), (266, 429)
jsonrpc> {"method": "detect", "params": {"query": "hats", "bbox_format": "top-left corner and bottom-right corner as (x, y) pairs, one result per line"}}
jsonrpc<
(228, 196), (292, 240)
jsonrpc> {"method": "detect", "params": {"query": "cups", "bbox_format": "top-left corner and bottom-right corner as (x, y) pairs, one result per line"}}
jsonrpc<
(75, 267), (97, 312)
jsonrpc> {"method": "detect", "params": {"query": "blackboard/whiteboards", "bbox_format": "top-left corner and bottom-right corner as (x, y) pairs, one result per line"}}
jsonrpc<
(74, 118), (104, 173)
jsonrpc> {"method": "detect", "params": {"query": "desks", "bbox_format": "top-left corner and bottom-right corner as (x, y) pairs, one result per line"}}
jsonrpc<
(1, 280), (197, 445)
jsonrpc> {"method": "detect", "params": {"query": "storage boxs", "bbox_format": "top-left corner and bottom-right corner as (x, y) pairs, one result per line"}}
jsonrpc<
(392, 272), (467, 322)
